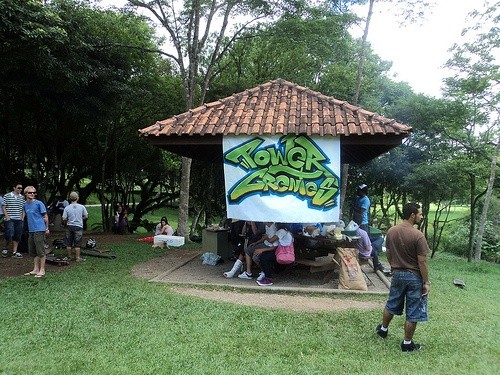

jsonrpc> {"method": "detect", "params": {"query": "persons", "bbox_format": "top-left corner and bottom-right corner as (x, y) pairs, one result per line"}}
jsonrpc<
(376, 203), (431, 352)
(112, 205), (138, 234)
(349, 184), (371, 234)
(62, 192), (89, 263)
(0, 190), (5, 236)
(349, 215), (384, 272)
(36, 196), (69, 226)
(154, 216), (175, 236)
(223, 219), (322, 286)
(1, 181), (26, 258)
(22, 186), (50, 277)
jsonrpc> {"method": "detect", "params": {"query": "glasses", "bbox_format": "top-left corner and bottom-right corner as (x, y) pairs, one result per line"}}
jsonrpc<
(15, 187), (22, 189)
(26, 192), (36, 194)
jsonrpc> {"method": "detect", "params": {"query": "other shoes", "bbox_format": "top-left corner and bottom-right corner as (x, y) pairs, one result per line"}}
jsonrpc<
(374, 262), (381, 268)
(76, 258), (84, 263)
(1, 248), (8, 258)
(401, 340), (421, 353)
(11, 252), (23, 258)
(375, 324), (388, 338)
(63, 257), (72, 261)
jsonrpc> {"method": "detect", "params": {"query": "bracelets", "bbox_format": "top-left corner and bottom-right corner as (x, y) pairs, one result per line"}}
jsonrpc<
(424, 281), (431, 285)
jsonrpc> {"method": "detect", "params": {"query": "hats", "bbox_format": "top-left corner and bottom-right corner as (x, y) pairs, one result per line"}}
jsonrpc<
(70, 192), (78, 199)
(357, 184), (368, 192)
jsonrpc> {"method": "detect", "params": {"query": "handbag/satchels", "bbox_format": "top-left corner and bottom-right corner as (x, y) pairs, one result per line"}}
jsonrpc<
(275, 243), (295, 264)
(52, 239), (67, 248)
(344, 219), (359, 231)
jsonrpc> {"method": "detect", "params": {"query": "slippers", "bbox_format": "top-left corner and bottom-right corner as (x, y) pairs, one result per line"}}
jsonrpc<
(35, 274), (44, 278)
(24, 272), (34, 276)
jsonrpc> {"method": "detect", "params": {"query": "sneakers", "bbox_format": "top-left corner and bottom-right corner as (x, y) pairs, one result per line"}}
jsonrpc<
(238, 271), (253, 280)
(256, 278), (272, 286)
(257, 271), (266, 281)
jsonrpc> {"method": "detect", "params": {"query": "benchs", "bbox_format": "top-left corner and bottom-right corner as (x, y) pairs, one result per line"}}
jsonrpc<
(296, 256), (326, 272)
